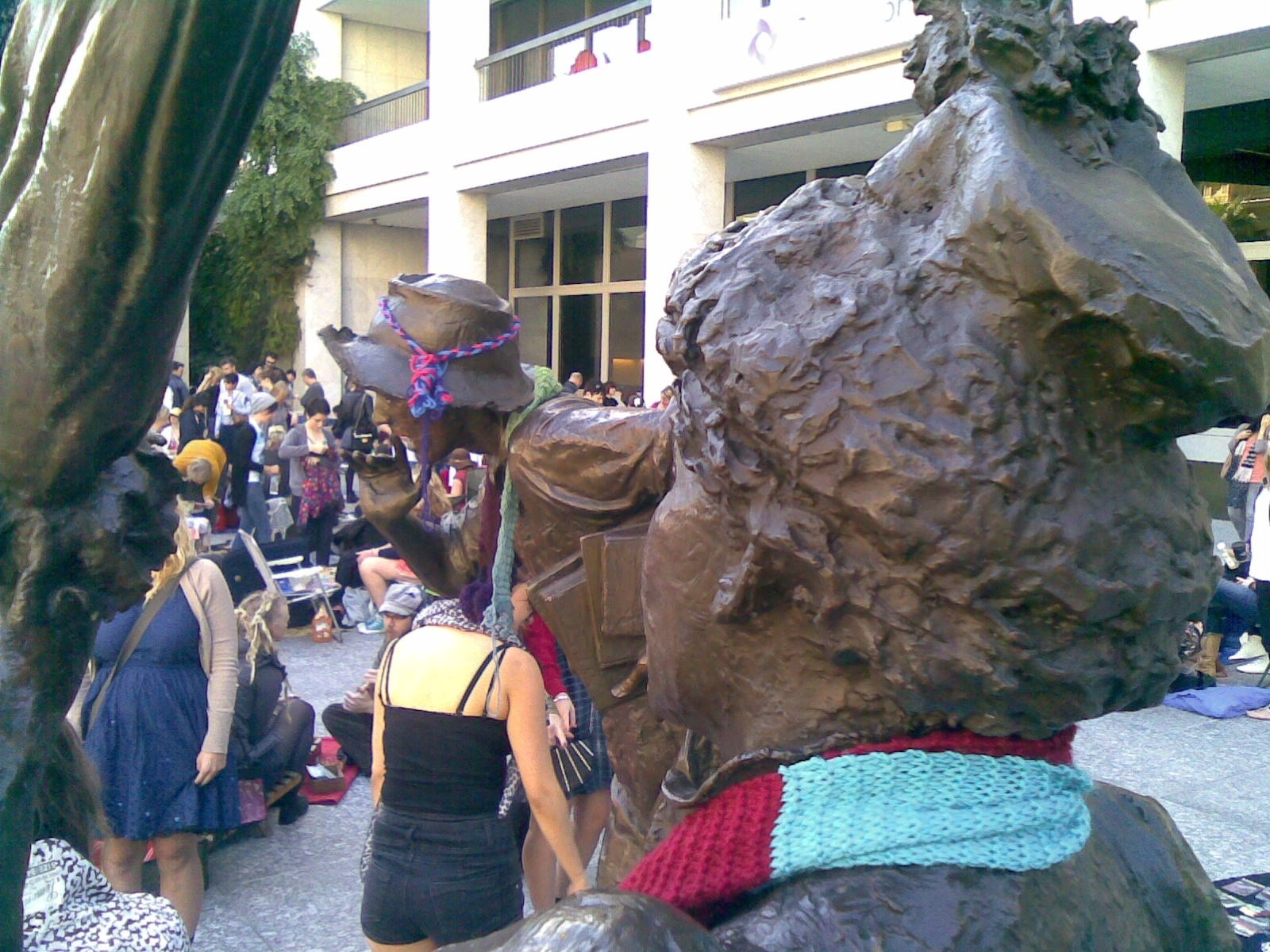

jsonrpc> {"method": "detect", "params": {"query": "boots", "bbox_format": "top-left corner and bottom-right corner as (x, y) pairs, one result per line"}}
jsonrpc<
(1191, 633), (1223, 679)
(346, 491), (359, 502)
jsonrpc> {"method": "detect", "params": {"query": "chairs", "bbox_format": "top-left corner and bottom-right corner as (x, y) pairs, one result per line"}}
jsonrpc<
(264, 555), (343, 645)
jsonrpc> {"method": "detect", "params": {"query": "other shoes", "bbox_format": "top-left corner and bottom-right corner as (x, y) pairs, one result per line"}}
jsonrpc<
(279, 795), (308, 825)
(333, 604), (344, 611)
(332, 623), (355, 631)
(336, 746), (353, 765)
(1247, 704), (1270, 720)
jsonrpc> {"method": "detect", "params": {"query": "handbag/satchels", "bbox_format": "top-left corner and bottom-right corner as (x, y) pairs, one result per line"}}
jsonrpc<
(341, 426), (375, 451)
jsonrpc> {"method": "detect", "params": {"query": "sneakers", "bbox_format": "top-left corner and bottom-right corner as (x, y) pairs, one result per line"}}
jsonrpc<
(1236, 653), (1270, 674)
(357, 613), (386, 634)
(1227, 632), (1267, 659)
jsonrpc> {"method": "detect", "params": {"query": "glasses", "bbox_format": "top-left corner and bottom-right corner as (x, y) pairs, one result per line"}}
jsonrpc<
(266, 360), (276, 365)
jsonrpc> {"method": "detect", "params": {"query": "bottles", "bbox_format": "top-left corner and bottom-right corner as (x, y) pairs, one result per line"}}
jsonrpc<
(269, 475), (279, 495)
(1217, 541), (1239, 570)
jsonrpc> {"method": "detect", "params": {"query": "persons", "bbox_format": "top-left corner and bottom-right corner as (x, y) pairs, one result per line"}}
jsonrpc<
(1177, 406), (1270, 719)
(23, 352), (681, 952)
(316, 273), (705, 891)
(437, 78), (1270, 952)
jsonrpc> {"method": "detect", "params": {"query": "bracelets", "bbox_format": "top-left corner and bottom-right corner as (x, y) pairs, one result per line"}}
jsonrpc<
(554, 695), (570, 703)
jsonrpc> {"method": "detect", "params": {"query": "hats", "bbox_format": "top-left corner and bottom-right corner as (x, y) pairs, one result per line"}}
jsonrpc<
(379, 583), (422, 616)
(251, 392), (276, 414)
(584, 378), (605, 390)
(195, 392), (212, 406)
(170, 407), (181, 416)
(446, 448), (474, 470)
(379, 430), (389, 442)
